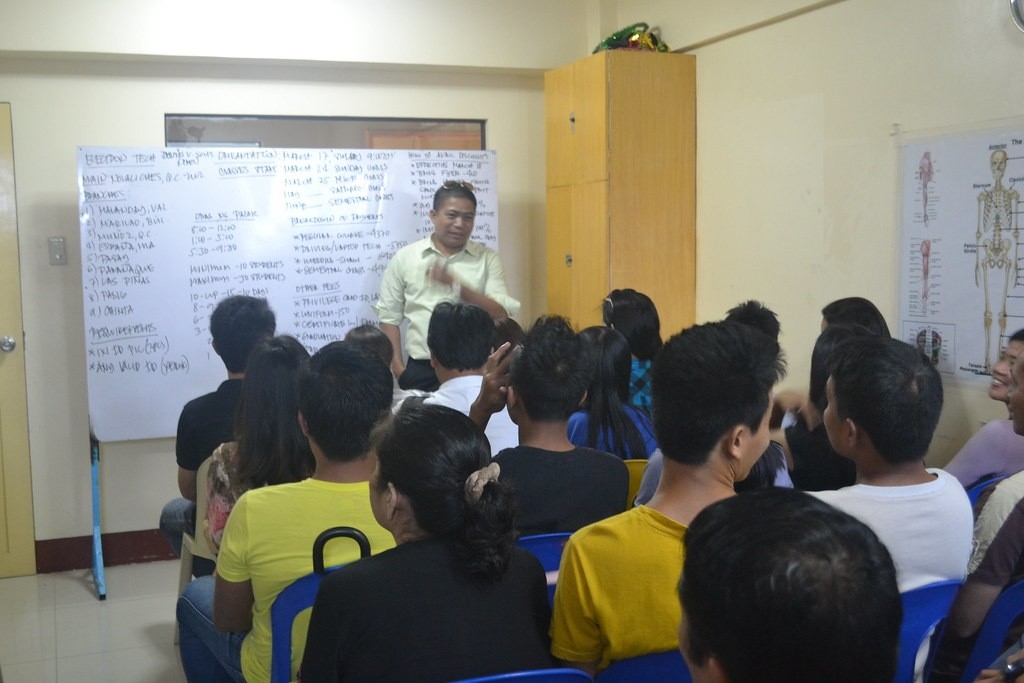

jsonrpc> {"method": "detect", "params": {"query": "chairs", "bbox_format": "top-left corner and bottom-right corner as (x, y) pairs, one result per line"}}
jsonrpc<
(269, 457), (1024, 683)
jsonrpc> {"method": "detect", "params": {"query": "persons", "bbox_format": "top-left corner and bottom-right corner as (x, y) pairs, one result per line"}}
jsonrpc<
(175, 340), (399, 683)
(158, 287), (974, 683)
(375, 181), (521, 391)
(295, 405), (549, 683)
(469, 314), (631, 537)
(674, 489), (903, 683)
(553, 323), (788, 677)
(942, 329), (1024, 683)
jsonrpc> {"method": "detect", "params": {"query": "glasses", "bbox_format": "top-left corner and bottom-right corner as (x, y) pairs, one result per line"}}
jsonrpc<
(439, 180), (474, 193)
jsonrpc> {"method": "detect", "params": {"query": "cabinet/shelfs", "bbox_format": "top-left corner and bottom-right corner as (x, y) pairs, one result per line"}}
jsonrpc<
(544, 49), (696, 339)
